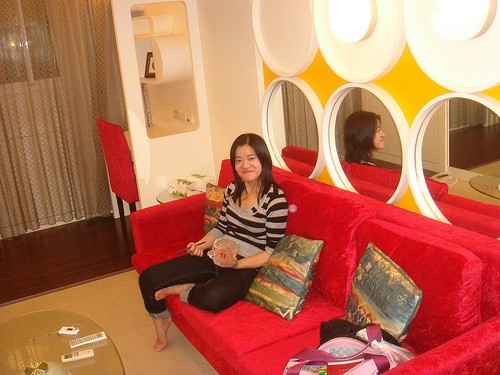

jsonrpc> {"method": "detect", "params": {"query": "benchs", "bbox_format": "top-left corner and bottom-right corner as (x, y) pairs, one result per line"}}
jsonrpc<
(281, 146), (500, 240)
(130, 160), (500, 375)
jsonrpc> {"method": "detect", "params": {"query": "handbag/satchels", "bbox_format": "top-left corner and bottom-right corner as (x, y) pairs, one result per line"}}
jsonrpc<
(279, 317), (415, 375)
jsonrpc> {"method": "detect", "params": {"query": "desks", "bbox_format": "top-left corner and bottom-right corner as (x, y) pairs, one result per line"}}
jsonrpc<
(156, 189), (185, 203)
(469, 174), (500, 199)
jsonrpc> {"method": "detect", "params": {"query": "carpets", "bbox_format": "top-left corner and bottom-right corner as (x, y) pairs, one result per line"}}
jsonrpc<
(0, 267), (219, 375)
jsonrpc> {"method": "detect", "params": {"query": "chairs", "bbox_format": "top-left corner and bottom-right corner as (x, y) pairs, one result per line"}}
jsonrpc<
(95, 119), (139, 245)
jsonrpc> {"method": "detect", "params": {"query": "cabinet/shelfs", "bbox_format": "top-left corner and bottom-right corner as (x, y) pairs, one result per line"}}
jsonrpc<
(130, 14), (191, 86)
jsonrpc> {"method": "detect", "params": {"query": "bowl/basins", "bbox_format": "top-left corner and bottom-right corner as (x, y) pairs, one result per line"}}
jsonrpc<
(212, 237), (240, 261)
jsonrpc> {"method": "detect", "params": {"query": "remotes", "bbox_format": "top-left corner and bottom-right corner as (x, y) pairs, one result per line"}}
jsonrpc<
(61, 349), (94, 362)
(69, 331), (107, 348)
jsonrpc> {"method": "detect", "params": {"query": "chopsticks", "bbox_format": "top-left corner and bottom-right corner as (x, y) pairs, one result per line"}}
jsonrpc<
(175, 241), (206, 254)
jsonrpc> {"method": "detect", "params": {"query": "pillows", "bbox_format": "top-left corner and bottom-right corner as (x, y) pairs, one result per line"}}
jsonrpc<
(345, 242), (422, 346)
(203, 182), (225, 236)
(241, 233), (324, 321)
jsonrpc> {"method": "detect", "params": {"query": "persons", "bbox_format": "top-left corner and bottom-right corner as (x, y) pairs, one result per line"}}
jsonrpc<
(343, 111), (385, 167)
(137, 133), (288, 351)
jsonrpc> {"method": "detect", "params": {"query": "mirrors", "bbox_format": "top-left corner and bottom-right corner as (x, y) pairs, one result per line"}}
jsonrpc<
(260, 75), (500, 241)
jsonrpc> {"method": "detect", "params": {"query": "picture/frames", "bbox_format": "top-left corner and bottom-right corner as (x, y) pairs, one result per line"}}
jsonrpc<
(144, 51), (156, 79)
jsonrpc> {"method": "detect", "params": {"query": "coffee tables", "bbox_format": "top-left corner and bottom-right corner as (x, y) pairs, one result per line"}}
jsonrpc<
(0, 309), (126, 375)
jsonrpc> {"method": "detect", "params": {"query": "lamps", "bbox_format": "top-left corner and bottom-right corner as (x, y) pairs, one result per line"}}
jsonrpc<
(429, 0), (497, 40)
(328, 0), (377, 46)
